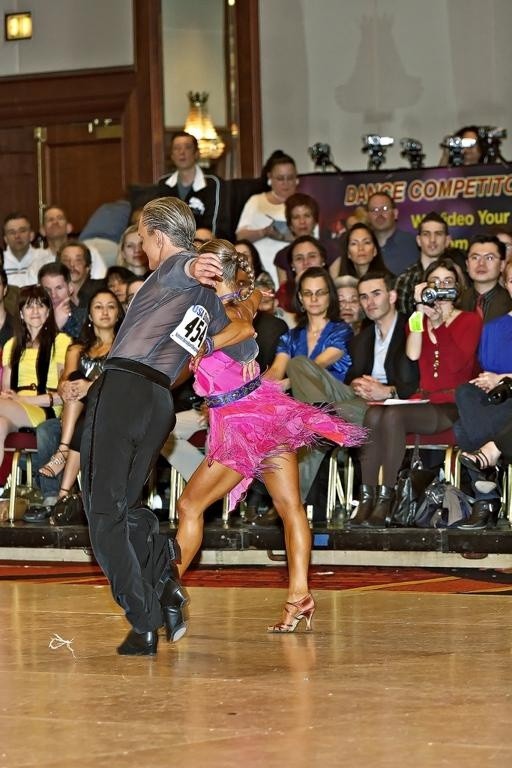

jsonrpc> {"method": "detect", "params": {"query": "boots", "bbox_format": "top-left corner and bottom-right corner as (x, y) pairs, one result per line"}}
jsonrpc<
(343, 483), (380, 528)
(360, 485), (396, 529)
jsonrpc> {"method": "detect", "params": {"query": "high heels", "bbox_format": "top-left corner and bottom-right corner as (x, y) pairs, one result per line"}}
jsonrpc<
(458, 448), (497, 481)
(474, 463), (504, 498)
(117, 629), (159, 657)
(265, 594), (316, 634)
(456, 497), (501, 531)
(159, 577), (191, 645)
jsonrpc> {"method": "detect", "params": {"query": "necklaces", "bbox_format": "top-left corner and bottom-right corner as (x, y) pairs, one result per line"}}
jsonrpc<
(271, 191), (288, 204)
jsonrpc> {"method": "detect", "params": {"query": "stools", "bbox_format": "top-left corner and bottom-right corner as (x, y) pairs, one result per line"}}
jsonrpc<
(0, 423), (511, 529)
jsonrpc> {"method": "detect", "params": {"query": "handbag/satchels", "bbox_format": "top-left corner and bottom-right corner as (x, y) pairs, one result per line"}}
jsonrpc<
(49, 493), (88, 527)
(387, 435), (436, 528)
(412, 476), (474, 528)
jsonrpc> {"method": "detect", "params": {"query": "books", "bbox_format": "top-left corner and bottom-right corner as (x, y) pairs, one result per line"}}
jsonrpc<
(269, 217), (294, 241)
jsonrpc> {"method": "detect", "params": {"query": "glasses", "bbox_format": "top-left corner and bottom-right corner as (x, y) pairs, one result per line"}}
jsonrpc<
(468, 254), (499, 264)
(303, 288), (329, 297)
(368, 206), (389, 215)
(427, 278), (456, 290)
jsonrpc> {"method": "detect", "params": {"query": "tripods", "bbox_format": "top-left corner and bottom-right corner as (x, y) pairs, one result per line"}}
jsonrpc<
(478, 145), (507, 165)
(314, 159), (341, 173)
(366, 153), (388, 170)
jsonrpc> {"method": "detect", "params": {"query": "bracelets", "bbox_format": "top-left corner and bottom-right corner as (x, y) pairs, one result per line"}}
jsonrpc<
(258, 229), (266, 238)
(281, 236), (285, 242)
(201, 334), (215, 359)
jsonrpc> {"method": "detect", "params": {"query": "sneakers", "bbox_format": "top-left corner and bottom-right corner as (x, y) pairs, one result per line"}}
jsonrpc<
(240, 499), (283, 528)
(22, 505), (56, 524)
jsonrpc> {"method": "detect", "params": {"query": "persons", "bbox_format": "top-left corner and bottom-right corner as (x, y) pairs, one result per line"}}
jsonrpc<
(434, 124), (492, 166)
(81, 196), (221, 658)
(169, 238), (314, 632)
(154, 132), (223, 242)
(233, 147), (320, 286)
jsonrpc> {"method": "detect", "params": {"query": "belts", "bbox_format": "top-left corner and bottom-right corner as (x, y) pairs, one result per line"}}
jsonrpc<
(102, 357), (172, 390)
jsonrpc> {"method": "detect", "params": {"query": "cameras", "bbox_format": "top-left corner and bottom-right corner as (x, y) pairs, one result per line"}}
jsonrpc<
(478, 126), (506, 145)
(421, 286), (458, 303)
(307, 142), (331, 159)
(400, 137), (423, 157)
(483, 377), (512, 404)
(440, 137), (476, 153)
(360, 134), (395, 153)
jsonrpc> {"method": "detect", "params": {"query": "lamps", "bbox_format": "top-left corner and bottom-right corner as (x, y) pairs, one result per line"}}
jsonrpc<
(183, 91), (226, 169)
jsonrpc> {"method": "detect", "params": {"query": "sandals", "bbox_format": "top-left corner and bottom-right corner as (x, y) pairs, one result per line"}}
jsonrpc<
(37, 442), (71, 480)
